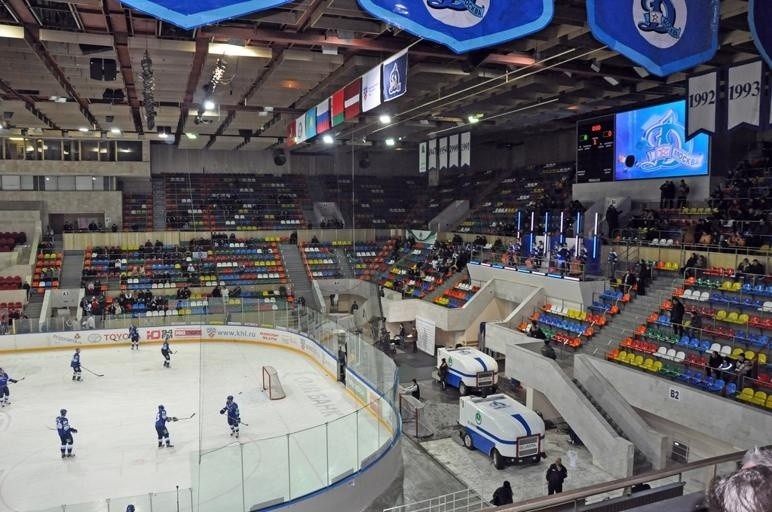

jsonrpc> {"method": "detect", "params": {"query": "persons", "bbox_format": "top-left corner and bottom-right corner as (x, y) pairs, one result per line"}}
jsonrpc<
(733, 258), (765, 287)
(546, 457), (567, 496)
(128, 324), (139, 350)
(542, 339), (557, 360)
(403, 378), (420, 398)
(0, 368), (17, 407)
(622, 258), (651, 295)
(71, 348), (81, 381)
(23, 281), (30, 300)
(694, 445), (772, 512)
(706, 351), (753, 386)
(80, 233), (359, 330)
(680, 253), (706, 281)
(528, 320), (546, 339)
(56, 408), (77, 458)
(606, 171), (770, 255)
(63, 219), (117, 233)
(161, 336), (172, 368)
(669, 297), (684, 337)
(394, 322), (406, 349)
(389, 234), (415, 261)
(438, 358), (448, 391)
(155, 405), (177, 447)
(308, 219), (343, 230)
(492, 237), (522, 266)
(393, 234), (487, 298)
(495, 174), (586, 276)
(687, 311), (701, 337)
(608, 247), (618, 278)
(412, 326), (418, 352)
(220, 396), (240, 438)
(493, 480), (513, 506)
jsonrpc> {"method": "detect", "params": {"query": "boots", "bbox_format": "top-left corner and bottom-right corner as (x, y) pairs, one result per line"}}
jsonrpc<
(164, 362), (170, 368)
(131, 345), (138, 350)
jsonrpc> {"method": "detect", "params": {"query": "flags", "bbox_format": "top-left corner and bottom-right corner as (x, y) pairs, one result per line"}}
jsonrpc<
(287, 47), (411, 147)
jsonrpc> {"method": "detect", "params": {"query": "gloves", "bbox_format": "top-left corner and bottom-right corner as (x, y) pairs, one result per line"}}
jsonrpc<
(220, 409), (225, 414)
(71, 429), (77, 433)
(167, 417), (179, 422)
(236, 417), (241, 423)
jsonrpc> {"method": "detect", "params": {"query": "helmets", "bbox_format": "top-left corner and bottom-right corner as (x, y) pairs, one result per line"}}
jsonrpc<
(60, 409), (68, 415)
(158, 405), (164, 410)
(227, 396), (233, 400)
(126, 505), (135, 511)
(76, 348), (81, 353)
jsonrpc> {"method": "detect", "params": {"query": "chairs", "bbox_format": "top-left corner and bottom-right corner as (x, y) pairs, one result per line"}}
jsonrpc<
(1, 172), (313, 332)
(519, 154), (772, 409)
(294, 159), (582, 325)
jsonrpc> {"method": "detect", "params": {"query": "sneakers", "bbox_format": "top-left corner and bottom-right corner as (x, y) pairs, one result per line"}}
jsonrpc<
(230, 431), (239, 439)
(1, 400), (11, 407)
(159, 443), (174, 448)
(62, 453), (75, 458)
(72, 376), (83, 381)
